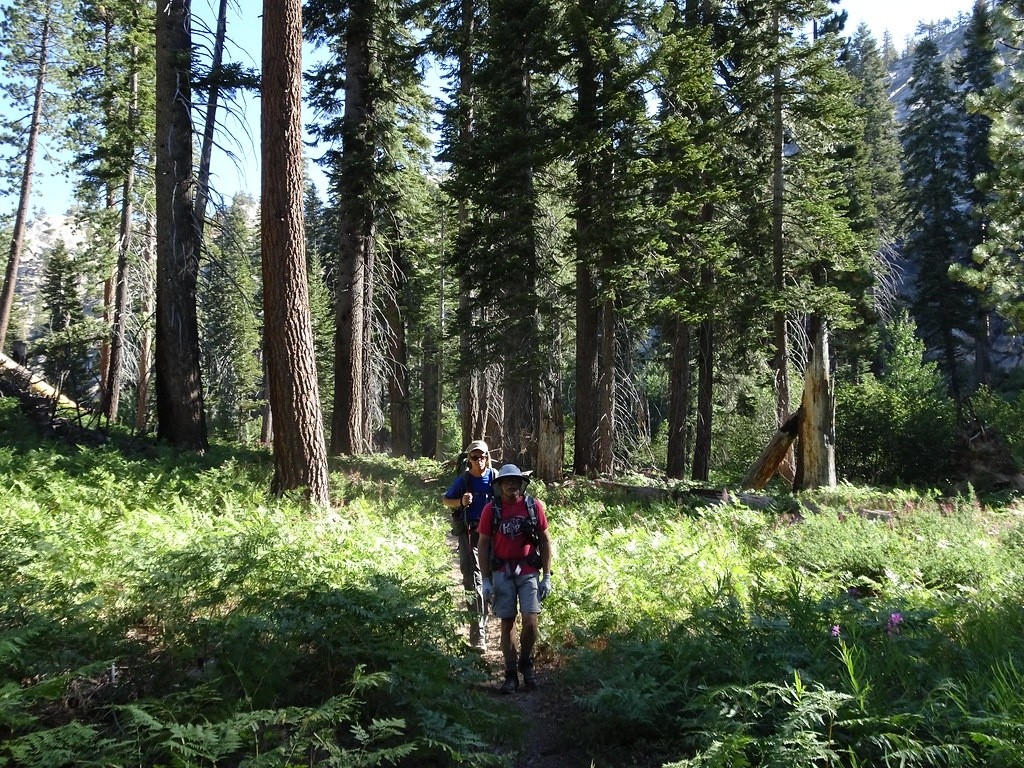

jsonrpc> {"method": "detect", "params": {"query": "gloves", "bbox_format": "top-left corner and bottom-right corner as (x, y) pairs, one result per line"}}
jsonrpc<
(539, 573), (551, 602)
(482, 578), (494, 605)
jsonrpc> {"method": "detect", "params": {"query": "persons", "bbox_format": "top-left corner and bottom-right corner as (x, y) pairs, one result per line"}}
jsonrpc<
(476, 464), (552, 694)
(442, 442), (499, 654)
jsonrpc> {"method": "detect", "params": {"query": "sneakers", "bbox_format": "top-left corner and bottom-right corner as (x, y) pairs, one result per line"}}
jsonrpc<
(518, 663), (538, 686)
(500, 672), (518, 693)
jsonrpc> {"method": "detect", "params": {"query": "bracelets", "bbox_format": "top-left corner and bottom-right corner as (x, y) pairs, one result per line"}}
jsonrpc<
(460, 498), (465, 508)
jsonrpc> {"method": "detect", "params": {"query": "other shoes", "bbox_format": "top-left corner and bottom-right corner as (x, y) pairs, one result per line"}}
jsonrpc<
(484, 624), (489, 642)
(473, 639), (488, 654)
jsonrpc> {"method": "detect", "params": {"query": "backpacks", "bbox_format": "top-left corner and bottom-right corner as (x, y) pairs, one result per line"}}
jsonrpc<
(451, 450), (499, 537)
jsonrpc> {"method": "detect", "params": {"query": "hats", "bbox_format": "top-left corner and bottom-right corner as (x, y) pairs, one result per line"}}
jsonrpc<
(492, 464), (530, 488)
(467, 440), (488, 455)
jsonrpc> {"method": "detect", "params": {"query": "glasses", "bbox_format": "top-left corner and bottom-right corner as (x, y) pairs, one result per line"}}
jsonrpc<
(469, 455), (486, 461)
